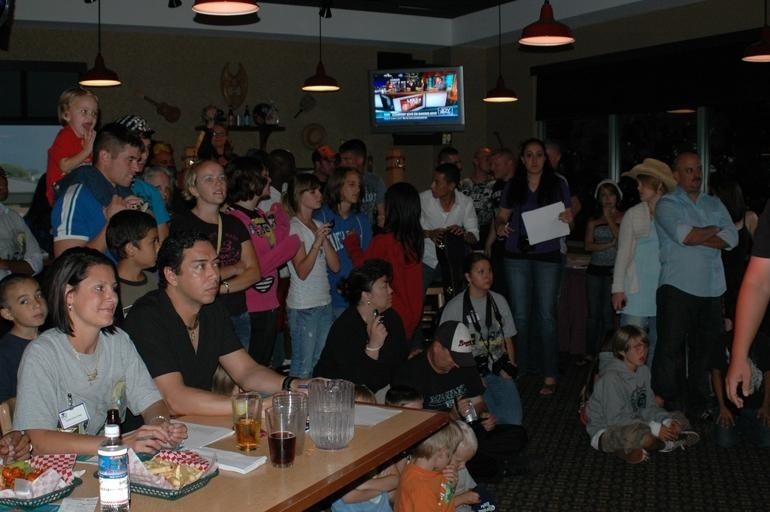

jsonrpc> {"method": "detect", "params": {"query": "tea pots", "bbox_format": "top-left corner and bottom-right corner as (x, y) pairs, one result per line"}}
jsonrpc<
(305, 377), (356, 451)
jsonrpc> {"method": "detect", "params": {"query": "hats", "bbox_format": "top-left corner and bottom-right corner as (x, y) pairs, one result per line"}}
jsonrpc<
(620, 157), (678, 192)
(594, 178), (623, 203)
(114, 113), (151, 139)
(312, 145), (338, 162)
(435, 321), (477, 368)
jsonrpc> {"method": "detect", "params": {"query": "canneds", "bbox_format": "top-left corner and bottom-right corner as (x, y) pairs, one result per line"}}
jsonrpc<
(464, 400), (478, 425)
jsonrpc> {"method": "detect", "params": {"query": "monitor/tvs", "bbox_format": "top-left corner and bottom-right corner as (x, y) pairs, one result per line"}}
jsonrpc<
(367, 65), (465, 134)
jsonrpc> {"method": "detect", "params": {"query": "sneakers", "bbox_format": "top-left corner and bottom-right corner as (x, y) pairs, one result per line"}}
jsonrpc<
(615, 448), (649, 465)
(658, 431), (701, 453)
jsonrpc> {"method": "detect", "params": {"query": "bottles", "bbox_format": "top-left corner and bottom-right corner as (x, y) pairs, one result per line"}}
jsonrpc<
(243, 105), (251, 126)
(227, 105), (235, 127)
(435, 476), (456, 511)
(96, 423), (133, 512)
(368, 154), (373, 172)
(105, 406), (121, 439)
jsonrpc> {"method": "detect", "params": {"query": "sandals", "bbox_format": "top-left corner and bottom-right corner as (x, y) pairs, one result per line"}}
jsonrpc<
(539, 381), (557, 398)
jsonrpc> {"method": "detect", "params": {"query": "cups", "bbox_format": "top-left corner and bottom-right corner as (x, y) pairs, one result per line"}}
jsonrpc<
(668, 415), (689, 439)
(264, 404), (299, 469)
(271, 391), (309, 454)
(230, 391), (263, 452)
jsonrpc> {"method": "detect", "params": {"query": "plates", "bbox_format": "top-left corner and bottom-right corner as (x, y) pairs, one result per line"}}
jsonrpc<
(301, 122), (327, 150)
(252, 102), (281, 126)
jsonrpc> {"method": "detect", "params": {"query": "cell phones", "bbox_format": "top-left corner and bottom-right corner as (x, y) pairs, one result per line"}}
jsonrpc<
(328, 218), (335, 234)
(616, 299), (625, 311)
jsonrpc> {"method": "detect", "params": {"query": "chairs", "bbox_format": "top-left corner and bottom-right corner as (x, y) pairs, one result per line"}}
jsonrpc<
(420, 287), (446, 348)
(1, 397), (17, 434)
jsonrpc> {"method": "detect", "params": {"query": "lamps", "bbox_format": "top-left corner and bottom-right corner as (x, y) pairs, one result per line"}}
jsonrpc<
(742, 0), (770, 64)
(480, 0), (520, 102)
(78, 0), (122, 87)
(300, 7), (341, 92)
(192, 0), (261, 17)
(518, 0), (576, 47)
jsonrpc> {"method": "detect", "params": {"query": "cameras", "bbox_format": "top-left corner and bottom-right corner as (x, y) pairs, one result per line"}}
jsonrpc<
(464, 400), (489, 428)
(475, 356), (490, 378)
(492, 351), (518, 378)
